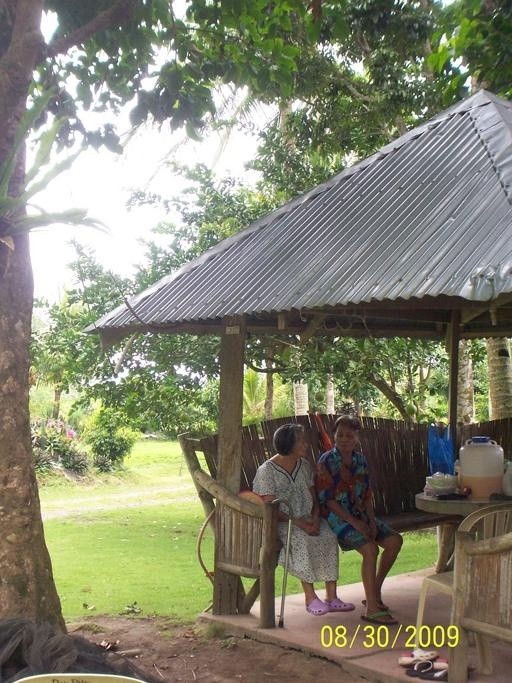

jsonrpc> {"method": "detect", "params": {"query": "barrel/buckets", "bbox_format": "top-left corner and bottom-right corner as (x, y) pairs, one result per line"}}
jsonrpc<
(458, 436), (505, 501)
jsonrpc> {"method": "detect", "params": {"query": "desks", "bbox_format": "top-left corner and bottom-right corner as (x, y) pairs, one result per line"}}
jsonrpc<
(415, 492), (512, 517)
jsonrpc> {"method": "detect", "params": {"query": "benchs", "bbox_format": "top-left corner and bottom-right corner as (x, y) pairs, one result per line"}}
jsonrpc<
(448, 501), (512, 683)
(177, 415), (512, 629)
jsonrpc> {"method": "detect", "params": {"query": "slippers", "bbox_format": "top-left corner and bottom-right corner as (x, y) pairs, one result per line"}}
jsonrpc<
(306, 598), (398, 624)
(399, 647), (477, 680)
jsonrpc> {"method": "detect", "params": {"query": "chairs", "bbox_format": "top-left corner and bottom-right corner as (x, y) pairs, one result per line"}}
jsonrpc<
(413, 506), (512, 683)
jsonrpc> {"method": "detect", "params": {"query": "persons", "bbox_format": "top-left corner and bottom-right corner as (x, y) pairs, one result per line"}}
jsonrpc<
(252, 423), (355, 616)
(315, 415), (403, 626)
